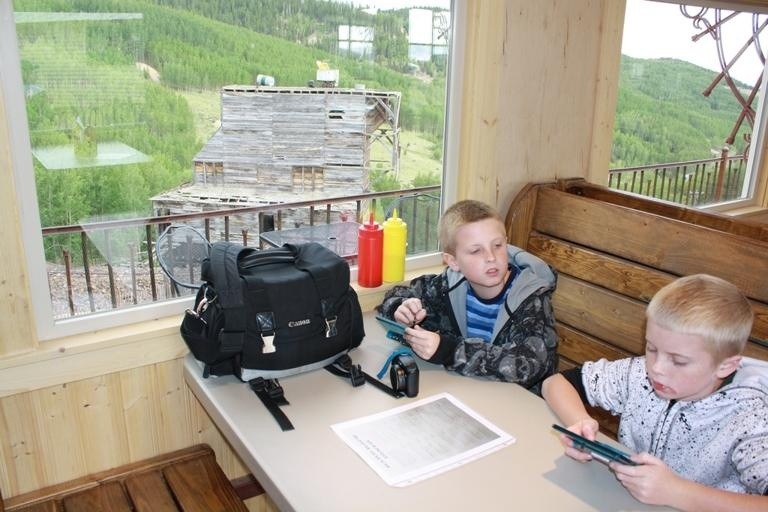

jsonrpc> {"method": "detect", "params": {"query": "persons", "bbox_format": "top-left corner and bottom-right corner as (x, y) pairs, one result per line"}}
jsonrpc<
(540, 272), (768, 511)
(382, 201), (559, 396)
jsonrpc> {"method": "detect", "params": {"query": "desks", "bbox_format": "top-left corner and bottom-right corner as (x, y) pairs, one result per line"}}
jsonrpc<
(257, 221), (362, 263)
(182, 310), (680, 512)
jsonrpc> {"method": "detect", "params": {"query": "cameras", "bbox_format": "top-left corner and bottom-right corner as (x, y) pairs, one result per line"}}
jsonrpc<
(390, 353), (419, 398)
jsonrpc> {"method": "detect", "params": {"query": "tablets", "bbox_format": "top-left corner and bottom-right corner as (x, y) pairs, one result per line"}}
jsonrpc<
(552, 423), (638, 466)
(374, 314), (411, 345)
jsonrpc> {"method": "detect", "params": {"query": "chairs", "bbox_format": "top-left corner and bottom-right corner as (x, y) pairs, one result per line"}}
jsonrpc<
(385, 194), (439, 256)
(154, 224), (212, 296)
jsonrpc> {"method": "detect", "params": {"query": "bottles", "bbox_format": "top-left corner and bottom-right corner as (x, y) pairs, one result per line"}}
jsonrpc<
(383, 208), (407, 284)
(358, 211), (383, 288)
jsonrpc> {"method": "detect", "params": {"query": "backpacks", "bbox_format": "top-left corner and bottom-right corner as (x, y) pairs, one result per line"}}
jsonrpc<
(181, 242), (364, 382)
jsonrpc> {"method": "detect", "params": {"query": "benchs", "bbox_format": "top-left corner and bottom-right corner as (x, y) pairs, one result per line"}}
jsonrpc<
(1, 442), (254, 511)
(503, 177), (767, 443)
